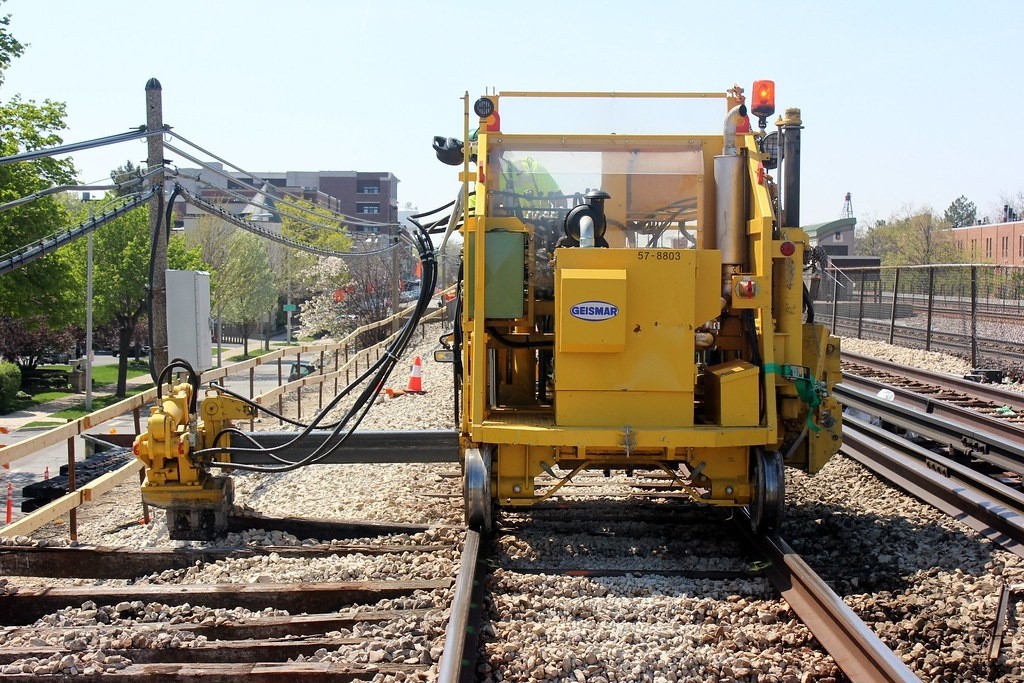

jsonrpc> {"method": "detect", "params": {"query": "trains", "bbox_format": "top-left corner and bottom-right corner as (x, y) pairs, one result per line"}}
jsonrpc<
(431, 78), (845, 535)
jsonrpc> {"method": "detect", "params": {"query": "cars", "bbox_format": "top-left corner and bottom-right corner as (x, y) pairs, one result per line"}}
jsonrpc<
(337, 314), (360, 324)
(399, 290), (420, 303)
(40, 345), (84, 366)
(112, 340), (141, 357)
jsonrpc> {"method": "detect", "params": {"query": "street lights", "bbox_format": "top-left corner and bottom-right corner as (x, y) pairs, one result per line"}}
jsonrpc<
(85, 190), (171, 410)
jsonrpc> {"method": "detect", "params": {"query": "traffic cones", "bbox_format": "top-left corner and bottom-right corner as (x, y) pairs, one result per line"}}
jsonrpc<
(402, 356), (427, 395)
